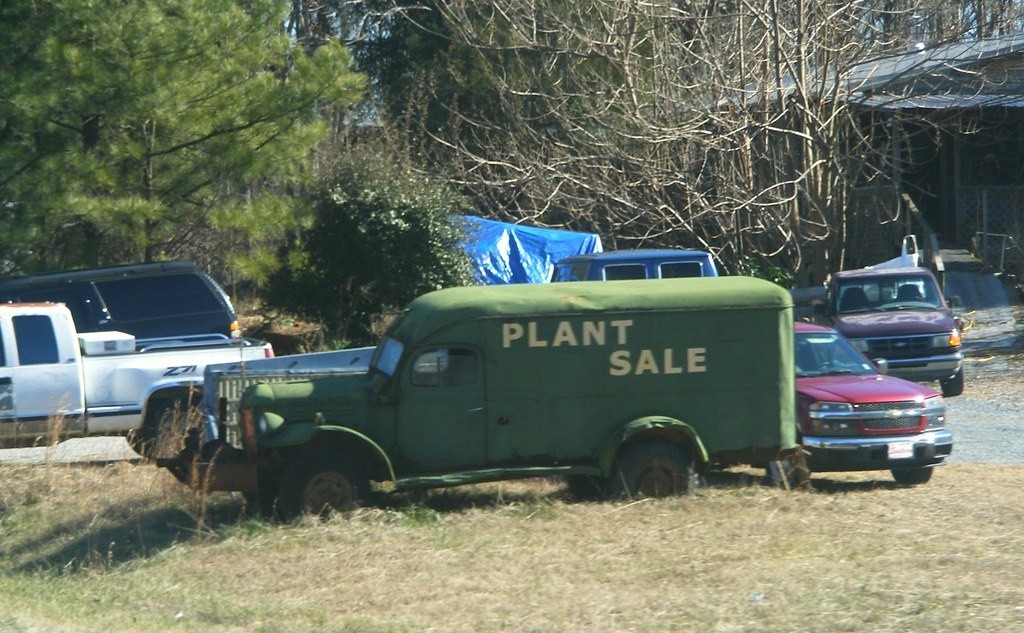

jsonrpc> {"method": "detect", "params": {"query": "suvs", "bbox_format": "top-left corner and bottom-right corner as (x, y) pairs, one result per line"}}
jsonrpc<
(821, 270), (965, 399)
(551, 248), (718, 282)
(121, 277), (796, 506)
(787, 322), (956, 484)
(1, 261), (241, 344)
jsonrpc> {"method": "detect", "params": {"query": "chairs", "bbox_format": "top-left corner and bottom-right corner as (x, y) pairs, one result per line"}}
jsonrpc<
(840, 287), (869, 310)
(897, 284), (923, 299)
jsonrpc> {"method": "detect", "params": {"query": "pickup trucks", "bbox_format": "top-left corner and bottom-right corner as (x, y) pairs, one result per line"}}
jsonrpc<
(0, 300), (278, 447)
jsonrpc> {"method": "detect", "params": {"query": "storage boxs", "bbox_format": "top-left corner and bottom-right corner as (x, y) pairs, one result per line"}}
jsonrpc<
(79, 331), (136, 356)
